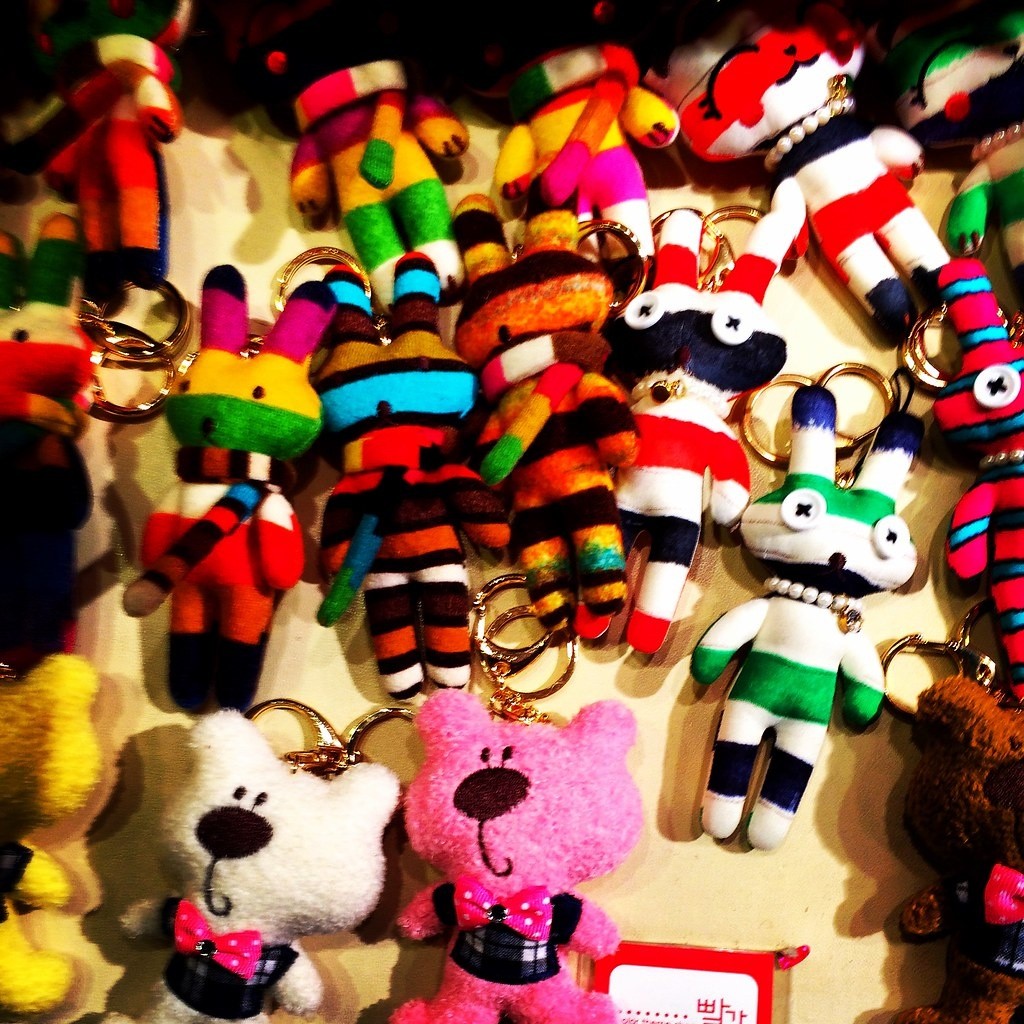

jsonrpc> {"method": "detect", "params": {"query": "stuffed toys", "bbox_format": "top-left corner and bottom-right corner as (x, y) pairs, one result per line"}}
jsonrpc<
(0, 0), (1024, 1024)
(689, 385), (919, 852)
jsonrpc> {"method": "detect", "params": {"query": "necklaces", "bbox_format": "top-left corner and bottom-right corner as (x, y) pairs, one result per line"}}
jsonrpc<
(971, 122), (1024, 162)
(980, 449), (1024, 467)
(759, 575), (864, 633)
(764, 77), (856, 170)
